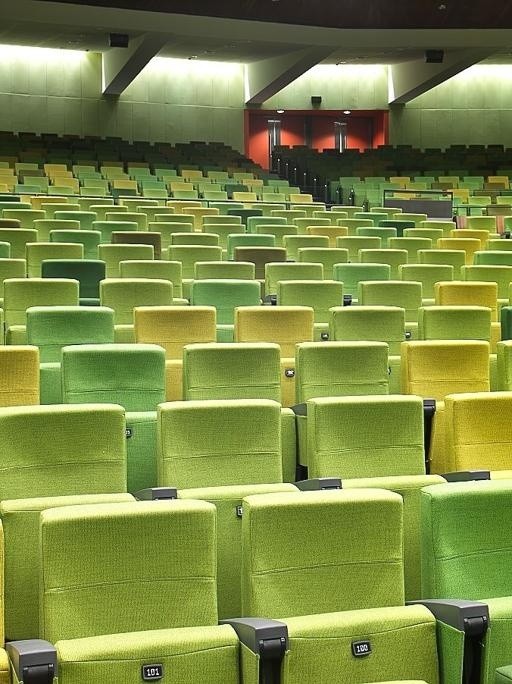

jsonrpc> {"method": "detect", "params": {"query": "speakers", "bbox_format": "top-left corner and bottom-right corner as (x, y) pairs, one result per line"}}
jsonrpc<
(109, 31), (129, 47)
(425, 49), (444, 63)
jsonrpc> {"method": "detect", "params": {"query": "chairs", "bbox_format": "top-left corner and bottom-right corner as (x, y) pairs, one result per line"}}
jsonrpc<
(0, 128), (512, 683)
